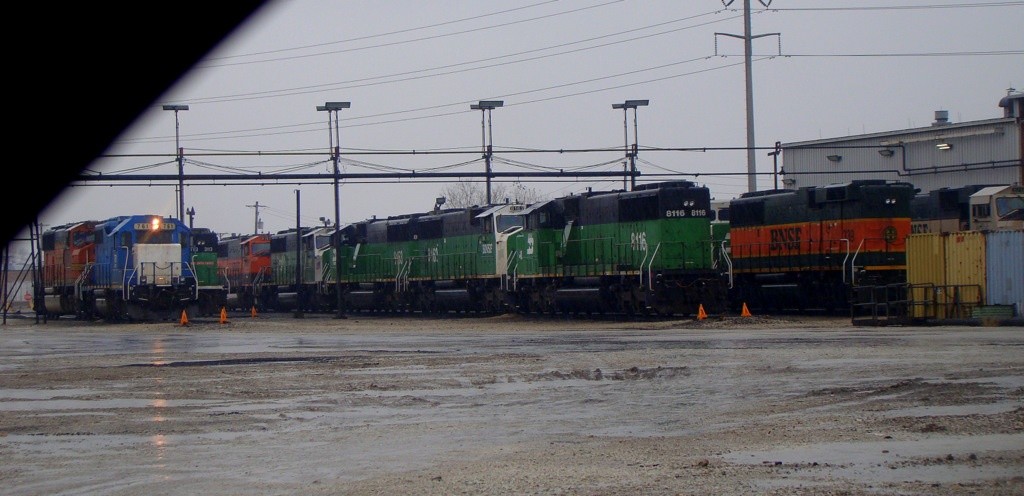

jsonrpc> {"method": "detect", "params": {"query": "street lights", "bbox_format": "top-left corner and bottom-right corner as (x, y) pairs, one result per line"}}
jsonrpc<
(320, 101), (350, 318)
(611, 98), (650, 191)
(161, 104), (186, 227)
(469, 99), (505, 206)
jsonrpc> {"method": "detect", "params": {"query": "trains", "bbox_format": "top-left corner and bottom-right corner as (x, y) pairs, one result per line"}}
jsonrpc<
(33, 180), (1024, 322)
